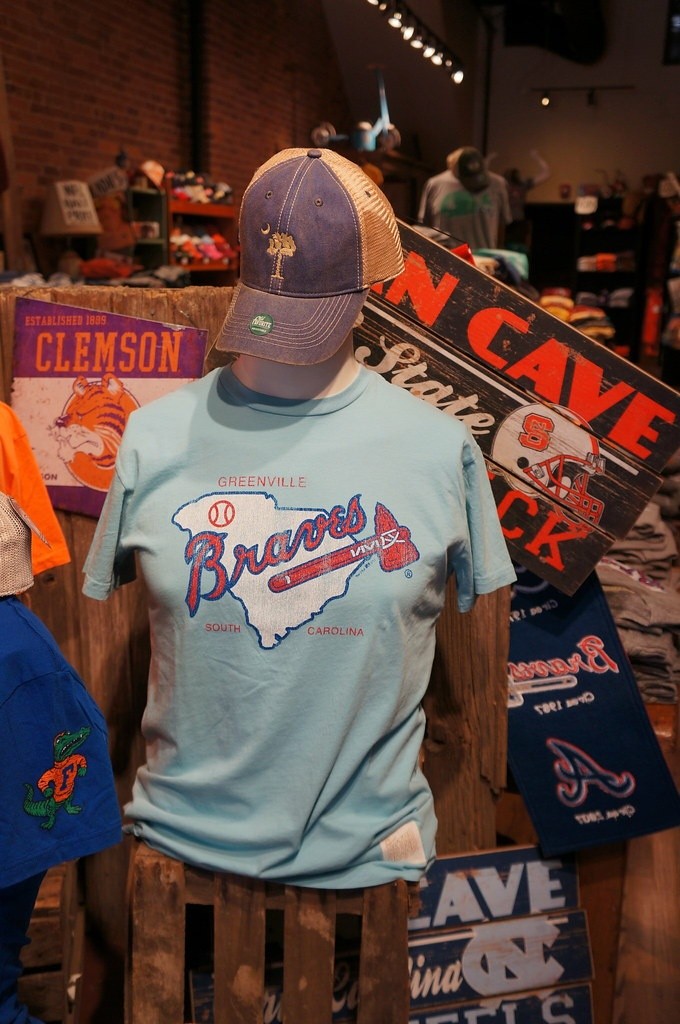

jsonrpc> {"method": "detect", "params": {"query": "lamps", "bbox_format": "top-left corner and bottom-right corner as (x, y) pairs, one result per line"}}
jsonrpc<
(367, 0), (464, 83)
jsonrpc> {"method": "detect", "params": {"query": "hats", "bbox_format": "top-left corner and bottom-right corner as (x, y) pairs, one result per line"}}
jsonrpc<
(215, 148), (406, 366)
(0, 491), (52, 597)
(448, 147), (490, 195)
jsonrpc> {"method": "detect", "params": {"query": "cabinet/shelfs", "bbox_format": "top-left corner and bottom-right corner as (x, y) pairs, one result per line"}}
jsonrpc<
(125, 178), (237, 287)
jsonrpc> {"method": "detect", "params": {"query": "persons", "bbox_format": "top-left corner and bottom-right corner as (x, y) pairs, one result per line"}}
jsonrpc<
(418, 148), (510, 250)
(77, 313), (516, 887)
(0, 403), (124, 1024)
